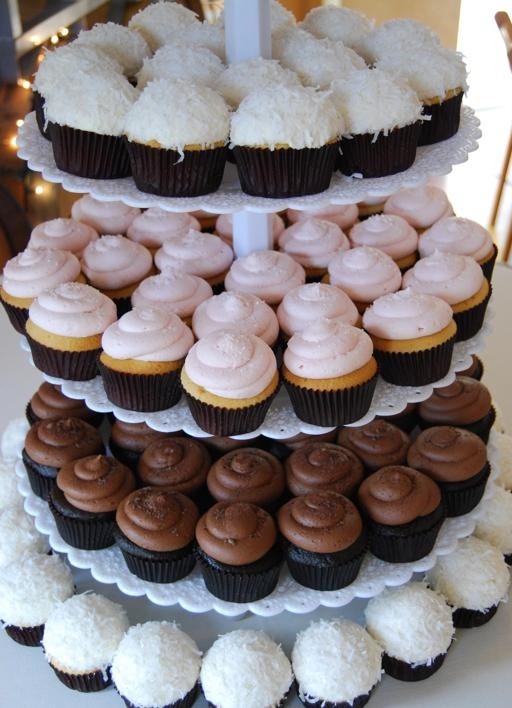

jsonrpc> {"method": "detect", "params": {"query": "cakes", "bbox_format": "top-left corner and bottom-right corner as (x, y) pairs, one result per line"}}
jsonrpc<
(363, 580), (456, 683)
(269, 26), (369, 92)
(356, 463), (447, 563)
(180, 329), (281, 438)
(401, 248), (494, 343)
(382, 184), (455, 235)
(276, 283), (363, 353)
(277, 218), (350, 283)
(26, 215), (97, 258)
(24, 282), (118, 383)
(223, 249), (306, 315)
(363, 286), (459, 387)
(24, 380), (105, 431)
(1, 246), (82, 337)
(131, 264), (213, 343)
(134, 44), (225, 90)
(372, 42), (470, 147)
(99, 305), (194, 415)
(195, 500), (283, 604)
(423, 535), (512, 628)
(46, 453), (138, 551)
(418, 374), (498, 446)
(0, 553), (77, 648)
(81, 234), (153, 319)
(364, 17), (440, 68)
(326, 69), (423, 179)
(213, 56), (304, 165)
(228, 83), (346, 199)
(69, 21), (152, 88)
(112, 485), (199, 584)
(275, 488), (369, 591)
(20, 417), (105, 503)
(108, 619), (204, 708)
(472, 485), (512, 566)
(349, 213), (420, 277)
(338, 419), (411, 478)
(418, 215), (499, 283)
(107, 420), (176, 469)
(127, 0), (203, 54)
(39, 588), (130, 692)
(163, 22), (226, 64)
(199, 628), (294, 708)
(42, 72), (141, 181)
(137, 435), (211, 494)
(322, 244), (403, 316)
(206, 446), (285, 512)
(154, 228), (234, 296)
(280, 316), (380, 428)
(214, 213), (286, 247)
(125, 207), (202, 256)
(287, 204), (360, 233)
(214, 1), (297, 39)
(285, 441), (363, 496)
(70, 192), (141, 235)
(407, 425), (491, 519)
(290, 617), (386, 708)
(124, 76), (231, 199)
(0, 505), (53, 566)
(191, 290), (280, 354)
(30, 44), (125, 143)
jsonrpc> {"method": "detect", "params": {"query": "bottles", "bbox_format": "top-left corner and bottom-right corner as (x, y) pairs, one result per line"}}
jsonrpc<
(298, 2), (378, 69)
